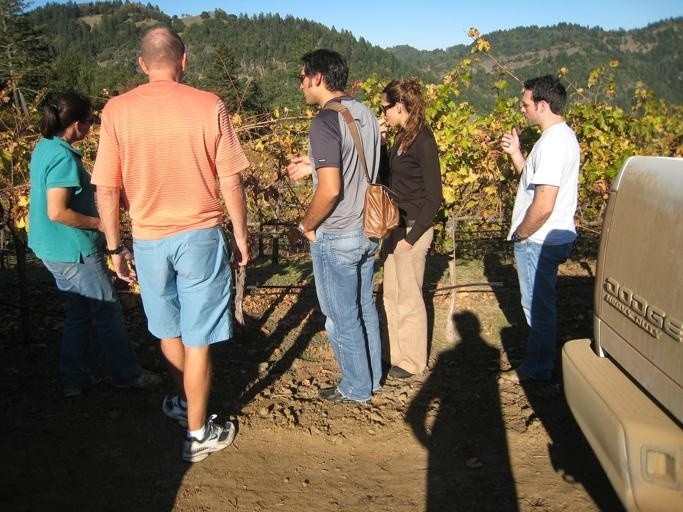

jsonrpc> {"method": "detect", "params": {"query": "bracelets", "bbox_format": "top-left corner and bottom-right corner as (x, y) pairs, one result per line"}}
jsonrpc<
(298, 221), (304, 233)
(510, 231), (522, 242)
(100, 243), (124, 255)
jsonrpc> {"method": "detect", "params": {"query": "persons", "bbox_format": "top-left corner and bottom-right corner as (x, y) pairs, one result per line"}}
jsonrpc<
(26, 90), (162, 398)
(89, 25), (250, 465)
(374, 79), (442, 380)
(496, 73), (581, 383)
(280, 47), (382, 404)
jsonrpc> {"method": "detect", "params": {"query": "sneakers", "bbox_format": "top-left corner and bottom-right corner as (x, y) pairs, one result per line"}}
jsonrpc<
(319, 387), (353, 403)
(162, 393), (189, 428)
(182, 418), (236, 463)
(114, 372), (162, 388)
(387, 366), (411, 380)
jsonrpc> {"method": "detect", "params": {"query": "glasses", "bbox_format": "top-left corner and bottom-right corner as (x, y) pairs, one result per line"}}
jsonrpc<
(379, 103), (396, 116)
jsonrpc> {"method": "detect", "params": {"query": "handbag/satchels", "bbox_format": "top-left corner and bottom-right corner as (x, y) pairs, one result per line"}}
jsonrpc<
(363, 181), (401, 240)
